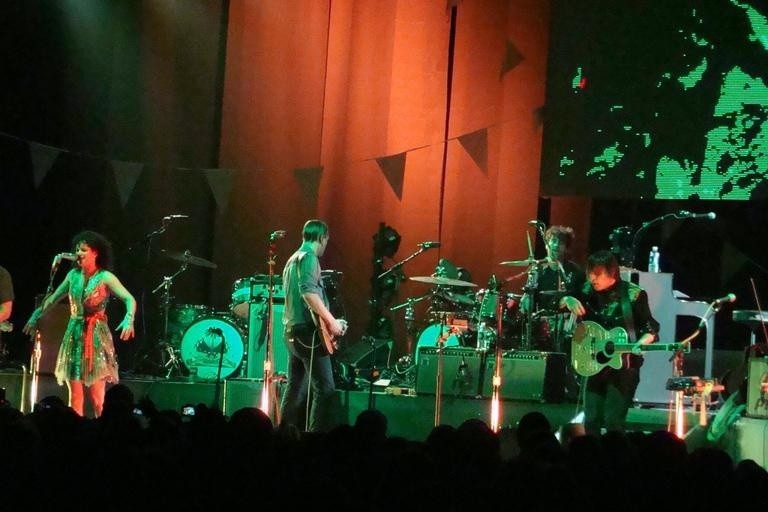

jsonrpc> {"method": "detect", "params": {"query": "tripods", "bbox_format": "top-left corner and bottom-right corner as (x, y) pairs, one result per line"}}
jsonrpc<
(127, 284), (185, 380)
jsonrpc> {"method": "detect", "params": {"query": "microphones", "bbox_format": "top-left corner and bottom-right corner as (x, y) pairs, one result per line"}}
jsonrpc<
(685, 211), (716, 222)
(60, 250), (81, 264)
(153, 214), (197, 221)
(715, 293), (737, 305)
(538, 256), (553, 264)
(416, 241), (442, 251)
(361, 335), (376, 351)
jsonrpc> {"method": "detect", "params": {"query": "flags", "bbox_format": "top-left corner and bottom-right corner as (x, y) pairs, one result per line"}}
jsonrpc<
(457, 126), (489, 180)
(108, 158), (147, 210)
(290, 165), (324, 219)
(374, 151), (411, 201)
(200, 167), (239, 217)
(27, 141), (61, 191)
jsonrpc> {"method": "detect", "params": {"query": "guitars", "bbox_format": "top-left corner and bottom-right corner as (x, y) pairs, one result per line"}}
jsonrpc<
(571, 321), (690, 376)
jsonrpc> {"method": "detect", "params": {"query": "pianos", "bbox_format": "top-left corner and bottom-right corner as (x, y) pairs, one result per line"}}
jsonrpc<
(620, 262), (717, 409)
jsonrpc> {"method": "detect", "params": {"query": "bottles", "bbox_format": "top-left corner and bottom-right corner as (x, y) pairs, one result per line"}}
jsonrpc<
(188, 363), (198, 384)
(649, 246), (660, 274)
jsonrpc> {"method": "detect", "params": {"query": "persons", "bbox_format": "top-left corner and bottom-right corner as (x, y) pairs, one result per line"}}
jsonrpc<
(279, 219), (344, 433)
(533, 225), (586, 316)
(0, 266), (14, 323)
(547, 249), (661, 436)
(22, 231), (138, 419)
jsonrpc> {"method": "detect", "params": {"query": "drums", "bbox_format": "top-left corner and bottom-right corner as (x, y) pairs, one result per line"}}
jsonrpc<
(232, 277), (250, 318)
(480, 291), (519, 325)
(532, 312), (557, 339)
(180, 317), (245, 381)
(414, 322), (467, 368)
(438, 292), (475, 312)
(175, 304), (209, 324)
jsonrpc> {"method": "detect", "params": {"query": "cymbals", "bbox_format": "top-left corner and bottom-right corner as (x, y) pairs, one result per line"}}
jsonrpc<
(500, 258), (547, 266)
(538, 288), (587, 299)
(161, 250), (217, 269)
(409, 276), (478, 287)
